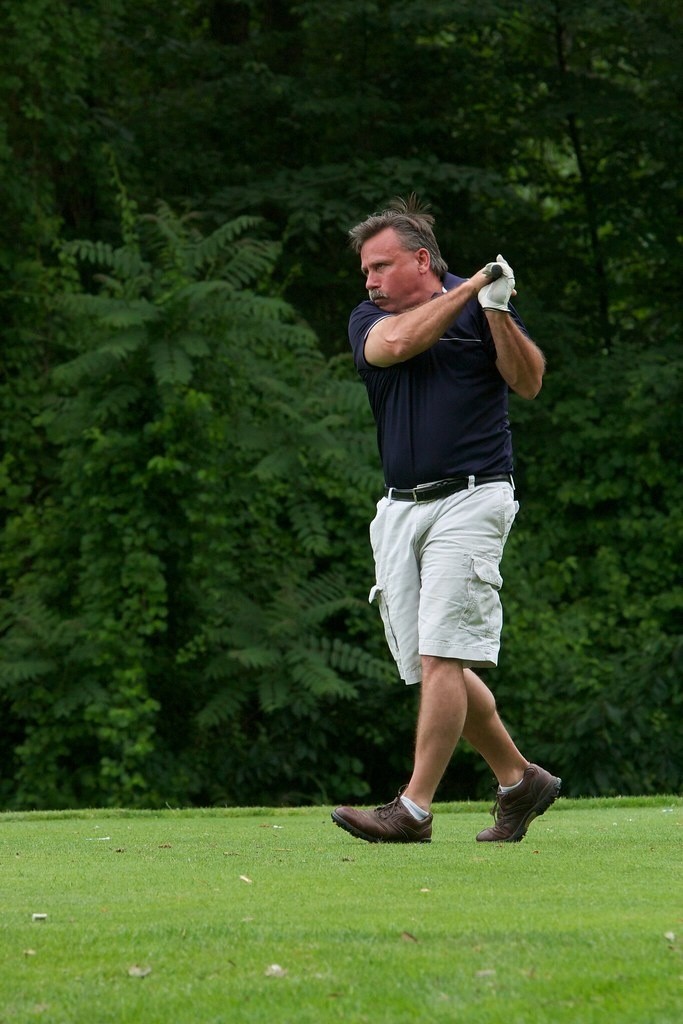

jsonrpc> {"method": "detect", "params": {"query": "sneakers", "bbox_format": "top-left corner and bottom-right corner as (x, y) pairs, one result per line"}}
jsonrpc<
(475, 763), (563, 844)
(329, 782), (434, 843)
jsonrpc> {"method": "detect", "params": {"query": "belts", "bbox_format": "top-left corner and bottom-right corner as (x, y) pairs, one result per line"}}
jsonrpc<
(383, 471), (510, 504)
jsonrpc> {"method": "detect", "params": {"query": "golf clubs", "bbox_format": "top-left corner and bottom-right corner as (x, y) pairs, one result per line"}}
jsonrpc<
(491, 264), (503, 277)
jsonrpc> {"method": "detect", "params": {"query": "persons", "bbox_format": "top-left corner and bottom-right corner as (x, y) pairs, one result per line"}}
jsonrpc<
(331, 190), (562, 843)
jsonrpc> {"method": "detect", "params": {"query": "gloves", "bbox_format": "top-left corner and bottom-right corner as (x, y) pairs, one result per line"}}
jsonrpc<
(478, 253), (516, 313)
